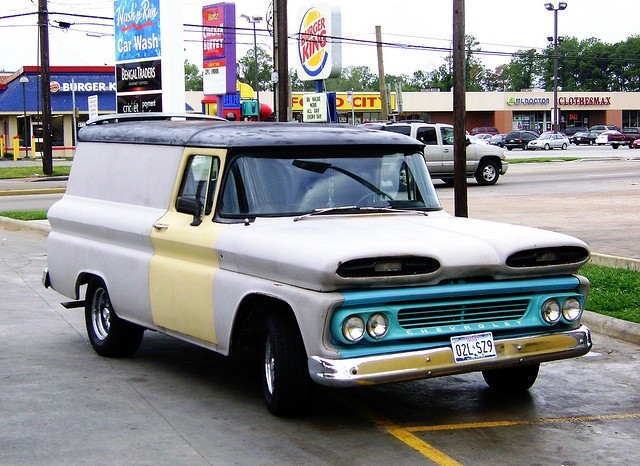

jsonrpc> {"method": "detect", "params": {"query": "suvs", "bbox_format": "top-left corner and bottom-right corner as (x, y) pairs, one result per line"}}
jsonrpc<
(505, 130), (539, 150)
(380, 118), (509, 192)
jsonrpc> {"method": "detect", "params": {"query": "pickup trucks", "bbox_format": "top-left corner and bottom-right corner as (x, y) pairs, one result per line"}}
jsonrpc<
(607, 126), (640, 149)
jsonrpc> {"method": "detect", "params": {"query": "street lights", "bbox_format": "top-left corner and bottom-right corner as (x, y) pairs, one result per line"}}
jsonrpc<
(19, 76), (30, 160)
(544, 1), (567, 133)
(238, 11), (264, 121)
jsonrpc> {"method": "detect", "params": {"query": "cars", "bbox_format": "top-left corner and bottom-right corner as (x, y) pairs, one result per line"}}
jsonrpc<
(490, 134), (508, 148)
(631, 139), (640, 149)
(565, 125), (623, 146)
(41, 111), (595, 421)
(473, 134), (493, 145)
(527, 133), (570, 150)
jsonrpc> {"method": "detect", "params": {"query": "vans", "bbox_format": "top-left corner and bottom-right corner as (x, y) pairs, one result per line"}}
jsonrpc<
(468, 124), (500, 136)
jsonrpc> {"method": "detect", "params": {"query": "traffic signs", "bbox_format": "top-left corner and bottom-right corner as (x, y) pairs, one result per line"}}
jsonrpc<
(88, 95), (98, 120)
